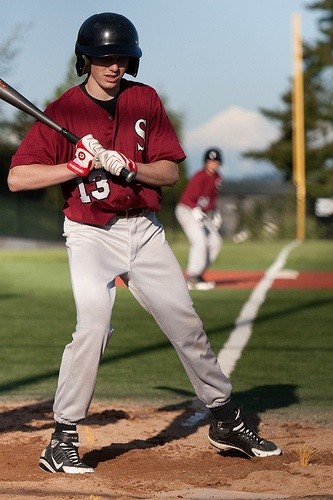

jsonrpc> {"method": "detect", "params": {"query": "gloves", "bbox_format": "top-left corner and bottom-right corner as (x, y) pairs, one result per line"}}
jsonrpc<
(65, 133), (107, 178)
(98, 149), (138, 182)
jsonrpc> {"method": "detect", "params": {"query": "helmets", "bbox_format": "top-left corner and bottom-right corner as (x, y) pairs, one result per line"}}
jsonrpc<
(74, 11), (143, 79)
(205, 149), (221, 161)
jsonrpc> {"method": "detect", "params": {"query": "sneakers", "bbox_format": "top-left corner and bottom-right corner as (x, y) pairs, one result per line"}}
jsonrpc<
(37, 432), (96, 476)
(204, 406), (283, 459)
(186, 276), (214, 291)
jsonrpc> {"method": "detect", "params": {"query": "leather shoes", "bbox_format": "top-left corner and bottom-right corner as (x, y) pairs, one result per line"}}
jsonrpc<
(119, 207), (144, 218)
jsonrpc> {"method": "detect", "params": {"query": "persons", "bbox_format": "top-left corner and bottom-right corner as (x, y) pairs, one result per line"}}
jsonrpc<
(7, 11), (282, 474)
(174, 148), (223, 291)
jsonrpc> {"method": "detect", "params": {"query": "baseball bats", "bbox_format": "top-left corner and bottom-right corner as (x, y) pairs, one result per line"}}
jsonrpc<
(0, 78), (139, 187)
